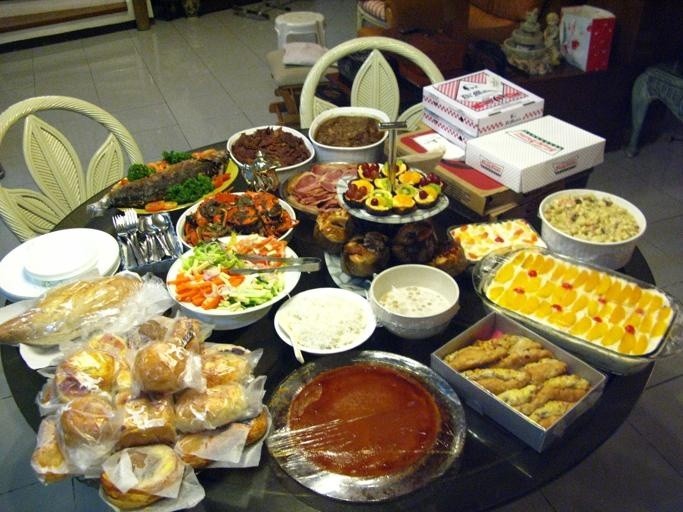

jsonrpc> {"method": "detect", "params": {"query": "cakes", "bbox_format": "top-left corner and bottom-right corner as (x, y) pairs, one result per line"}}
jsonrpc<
(314, 208), (357, 255)
(429, 241), (467, 276)
(343, 158), (447, 216)
(343, 230), (389, 279)
(450, 218), (547, 262)
(390, 219), (439, 262)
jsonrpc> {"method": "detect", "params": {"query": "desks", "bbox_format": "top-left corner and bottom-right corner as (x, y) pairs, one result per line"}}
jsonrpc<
(469, 41), (613, 153)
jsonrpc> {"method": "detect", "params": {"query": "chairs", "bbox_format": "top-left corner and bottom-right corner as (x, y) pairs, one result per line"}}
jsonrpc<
(626, 63), (683, 158)
(0, 95), (144, 244)
(356, 0), (545, 89)
(298, 36), (445, 129)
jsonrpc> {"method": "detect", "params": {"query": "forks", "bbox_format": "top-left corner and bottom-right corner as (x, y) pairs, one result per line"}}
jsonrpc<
(112, 209), (147, 269)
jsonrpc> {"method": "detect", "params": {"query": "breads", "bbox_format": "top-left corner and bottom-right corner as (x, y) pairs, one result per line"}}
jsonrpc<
(30, 317), (268, 511)
(444, 335), (592, 430)
(1, 276), (143, 348)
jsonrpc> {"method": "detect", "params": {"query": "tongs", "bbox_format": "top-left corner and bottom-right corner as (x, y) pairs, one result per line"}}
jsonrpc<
(232, 255), (320, 275)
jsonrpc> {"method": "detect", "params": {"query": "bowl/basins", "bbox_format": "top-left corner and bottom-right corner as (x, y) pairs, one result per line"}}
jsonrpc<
(370, 261), (460, 339)
(536, 187), (647, 269)
(227, 123), (314, 187)
(308, 107), (391, 164)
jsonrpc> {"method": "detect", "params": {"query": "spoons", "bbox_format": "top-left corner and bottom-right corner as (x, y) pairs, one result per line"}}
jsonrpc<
(138, 211), (178, 263)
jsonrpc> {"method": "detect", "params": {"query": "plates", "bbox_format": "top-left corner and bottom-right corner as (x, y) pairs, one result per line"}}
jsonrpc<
(271, 287), (378, 355)
(0, 226), (121, 300)
(260, 351), (465, 502)
(166, 232), (303, 317)
(323, 247), (367, 295)
(175, 193), (297, 248)
(110, 153), (236, 214)
(336, 174), (448, 222)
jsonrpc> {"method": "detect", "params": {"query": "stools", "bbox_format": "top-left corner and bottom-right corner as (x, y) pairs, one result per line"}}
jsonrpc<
(274, 11), (327, 50)
(266, 41), (339, 127)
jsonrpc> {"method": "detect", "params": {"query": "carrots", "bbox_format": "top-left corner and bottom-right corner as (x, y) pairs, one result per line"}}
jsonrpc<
(167, 235), (288, 310)
